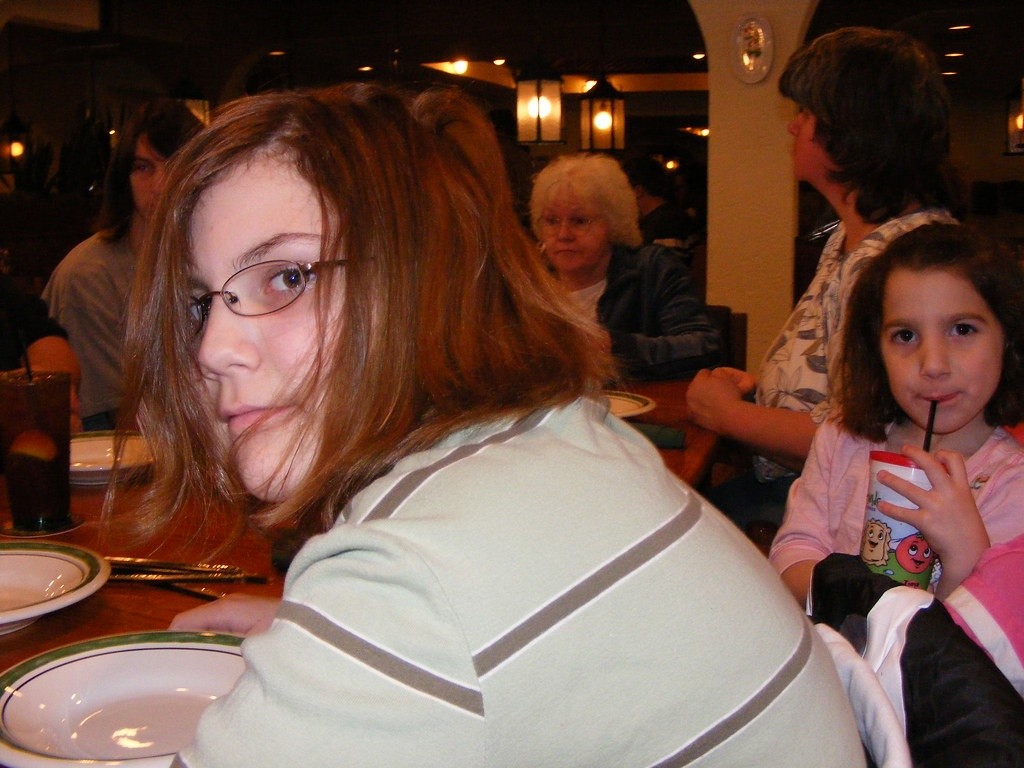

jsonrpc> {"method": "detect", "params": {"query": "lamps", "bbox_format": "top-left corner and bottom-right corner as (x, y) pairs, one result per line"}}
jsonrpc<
(514, 51), (567, 146)
(1002, 82), (1024, 156)
(578, 77), (626, 161)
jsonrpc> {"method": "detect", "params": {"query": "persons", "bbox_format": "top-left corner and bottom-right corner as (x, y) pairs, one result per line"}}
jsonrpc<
(99, 86), (866, 767)
(530, 155), (734, 384)
(622, 156), (704, 264)
(769, 220), (1024, 610)
(687, 29), (959, 550)
(0, 95), (192, 474)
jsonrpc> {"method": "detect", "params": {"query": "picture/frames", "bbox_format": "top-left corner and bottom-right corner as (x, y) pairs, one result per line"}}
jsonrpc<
(727, 13), (774, 84)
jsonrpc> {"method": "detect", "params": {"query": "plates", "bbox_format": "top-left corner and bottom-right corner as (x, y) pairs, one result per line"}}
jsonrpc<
(0, 522), (86, 538)
(604, 390), (655, 417)
(0, 630), (245, 768)
(0, 541), (111, 635)
(69, 430), (155, 485)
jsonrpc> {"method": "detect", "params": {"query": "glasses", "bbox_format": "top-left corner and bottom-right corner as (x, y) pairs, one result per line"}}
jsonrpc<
(187, 260), (347, 339)
(540, 212), (604, 237)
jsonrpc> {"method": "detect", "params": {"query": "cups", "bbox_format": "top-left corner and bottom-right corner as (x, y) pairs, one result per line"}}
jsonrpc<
(859, 450), (936, 590)
(0, 369), (71, 530)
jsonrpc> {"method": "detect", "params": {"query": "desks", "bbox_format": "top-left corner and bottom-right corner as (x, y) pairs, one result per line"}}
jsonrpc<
(0, 375), (725, 768)
